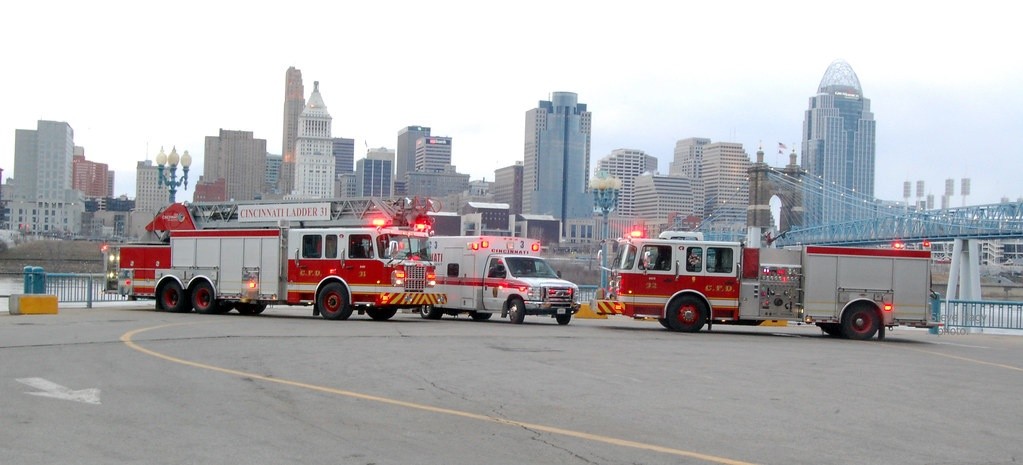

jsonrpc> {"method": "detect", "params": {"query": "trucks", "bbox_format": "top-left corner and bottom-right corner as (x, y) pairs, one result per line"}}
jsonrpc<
(396, 234), (581, 325)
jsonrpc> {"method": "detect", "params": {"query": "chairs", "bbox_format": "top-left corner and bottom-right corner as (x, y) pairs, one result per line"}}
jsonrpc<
(306, 242), (319, 258)
(652, 254), (665, 269)
(352, 238), (367, 258)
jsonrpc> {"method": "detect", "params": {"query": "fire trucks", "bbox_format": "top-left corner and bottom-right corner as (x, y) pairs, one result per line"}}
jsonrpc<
(593, 224), (945, 339)
(101, 194), (448, 320)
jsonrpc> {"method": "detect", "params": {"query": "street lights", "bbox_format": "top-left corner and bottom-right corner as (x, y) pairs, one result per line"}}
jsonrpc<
(589, 175), (622, 288)
(156, 145), (192, 204)
